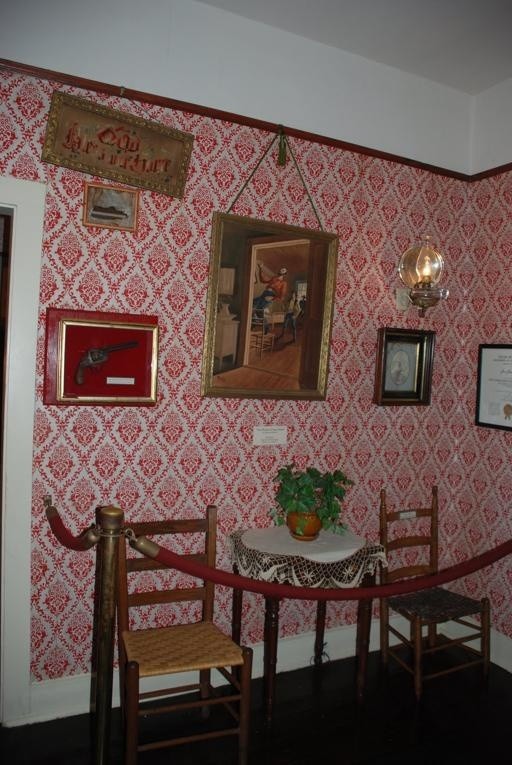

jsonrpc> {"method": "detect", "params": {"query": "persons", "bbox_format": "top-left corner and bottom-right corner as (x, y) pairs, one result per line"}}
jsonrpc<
(281, 290), (299, 343)
(298, 294), (306, 318)
(250, 262), (289, 336)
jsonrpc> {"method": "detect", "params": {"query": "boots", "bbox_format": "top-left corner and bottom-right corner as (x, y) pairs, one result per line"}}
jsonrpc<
(279, 328), (287, 338)
(290, 329), (296, 342)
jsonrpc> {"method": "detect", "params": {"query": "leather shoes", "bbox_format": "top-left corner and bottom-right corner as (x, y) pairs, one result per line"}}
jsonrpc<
(264, 328), (269, 335)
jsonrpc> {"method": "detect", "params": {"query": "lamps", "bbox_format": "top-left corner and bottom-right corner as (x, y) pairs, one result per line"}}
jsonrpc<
(397, 232), (449, 318)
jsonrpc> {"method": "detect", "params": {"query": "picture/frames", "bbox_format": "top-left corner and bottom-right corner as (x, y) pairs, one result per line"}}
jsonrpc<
(474, 343), (511, 432)
(200, 210), (340, 401)
(371, 327), (434, 406)
(83, 183), (139, 234)
(45, 306), (159, 406)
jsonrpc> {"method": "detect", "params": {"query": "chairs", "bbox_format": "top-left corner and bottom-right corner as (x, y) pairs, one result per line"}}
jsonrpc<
(380, 486), (491, 701)
(116, 505), (253, 765)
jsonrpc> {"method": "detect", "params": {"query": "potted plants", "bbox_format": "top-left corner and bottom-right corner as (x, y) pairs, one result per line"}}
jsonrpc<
(266, 461), (355, 541)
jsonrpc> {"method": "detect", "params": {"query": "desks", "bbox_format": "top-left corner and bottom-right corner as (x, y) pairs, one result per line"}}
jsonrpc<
(230, 526), (377, 730)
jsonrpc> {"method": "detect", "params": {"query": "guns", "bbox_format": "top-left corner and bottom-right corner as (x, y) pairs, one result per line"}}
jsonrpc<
(75, 340), (138, 385)
